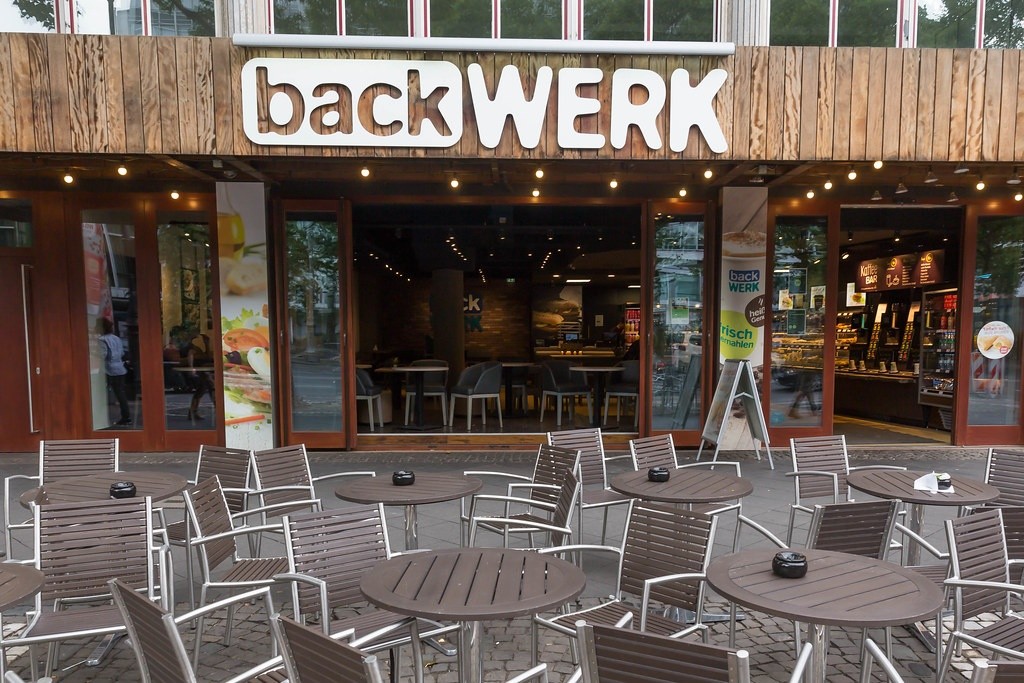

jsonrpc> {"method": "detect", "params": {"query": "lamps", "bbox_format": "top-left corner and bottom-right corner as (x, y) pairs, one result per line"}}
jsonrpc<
(847, 230), (853, 242)
(840, 247), (850, 261)
(953, 162), (970, 174)
(895, 179), (909, 194)
(942, 232), (948, 242)
(947, 190), (958, 203)
(924, 166), (938, 183)
(870, 188), (883, 201)
(1006, 166), (1021, 185)
(892, 228), (900, 242)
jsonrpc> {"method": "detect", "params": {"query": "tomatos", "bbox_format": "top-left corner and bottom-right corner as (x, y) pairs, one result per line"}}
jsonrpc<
(222, 328), (269, 351)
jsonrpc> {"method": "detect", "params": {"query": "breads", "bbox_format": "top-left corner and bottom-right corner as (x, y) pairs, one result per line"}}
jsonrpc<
(532, 299), (580, 327)
(219, 257), (267, 295)
(977, 335), (1011, 350)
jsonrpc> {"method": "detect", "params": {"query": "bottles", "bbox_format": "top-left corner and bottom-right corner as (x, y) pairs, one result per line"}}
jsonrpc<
(624, 310), (641, 353)
(931, 287), (1002, 393)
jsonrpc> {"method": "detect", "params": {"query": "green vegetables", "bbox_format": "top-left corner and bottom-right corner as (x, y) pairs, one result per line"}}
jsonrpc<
(220, 308), (271, 414)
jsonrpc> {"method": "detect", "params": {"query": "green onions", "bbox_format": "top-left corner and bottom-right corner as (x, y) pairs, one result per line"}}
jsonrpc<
(222, 322), (270, 430)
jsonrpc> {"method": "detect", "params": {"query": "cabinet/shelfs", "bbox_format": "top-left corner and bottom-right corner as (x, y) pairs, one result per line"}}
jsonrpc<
(916, 287), (1021, 430)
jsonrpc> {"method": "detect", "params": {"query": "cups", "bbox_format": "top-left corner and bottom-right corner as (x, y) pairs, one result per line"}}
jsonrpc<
(720, 254), (766, 369)
(859, 360), (865, 369)
(217, 211), (246, 260)
(879, 362), (886, 372)
(890, 362), (897, 371)
(849, 360), (856, 369)
(913, 363), (919, 374)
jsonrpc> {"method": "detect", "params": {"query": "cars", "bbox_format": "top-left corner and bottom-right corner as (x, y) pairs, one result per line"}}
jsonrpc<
(777, 367), (823, 392)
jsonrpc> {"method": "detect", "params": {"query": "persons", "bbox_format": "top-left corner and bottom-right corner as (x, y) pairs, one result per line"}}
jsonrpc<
(599, 320), (641, 405)
(609, 317), (624, 347)
(188, 333), (216, 420)
(788, 372), (821, 419)
(93, 318), (132, 426)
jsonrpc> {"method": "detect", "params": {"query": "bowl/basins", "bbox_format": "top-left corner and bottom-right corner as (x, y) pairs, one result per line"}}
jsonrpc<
(937, 475), (951, 490)
(648, 467), (670, 482)
(393, 471), (415, 485)
(772, 551), (808, 578)
(223, 371), (272, 403)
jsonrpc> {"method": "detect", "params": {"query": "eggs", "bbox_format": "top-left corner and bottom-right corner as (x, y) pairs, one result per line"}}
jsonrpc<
(246, 346), (271, 384)
(244, 316), (269, 343)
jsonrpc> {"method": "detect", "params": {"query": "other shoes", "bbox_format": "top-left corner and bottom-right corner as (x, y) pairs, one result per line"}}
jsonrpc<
(788, 410), (802, 419)
(812, 410), (818, 416)
(188, 410), (206, 421)
(113, 418), (132, 425)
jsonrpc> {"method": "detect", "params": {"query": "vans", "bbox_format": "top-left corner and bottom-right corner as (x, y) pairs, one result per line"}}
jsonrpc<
(667, 330), (702, 362)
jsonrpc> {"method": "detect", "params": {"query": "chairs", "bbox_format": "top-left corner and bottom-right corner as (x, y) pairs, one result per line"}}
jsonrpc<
(602, 359), (641, 427)
(448, 360), (504, 431)
(539, 358), (594, 425)
(0, 428), (1024, 683)
(403, 358), (449, 426)
(356, 368), (385, 431)
(497, 356), (531, 414)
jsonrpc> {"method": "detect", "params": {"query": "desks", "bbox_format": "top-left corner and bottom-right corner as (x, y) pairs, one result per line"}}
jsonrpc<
(356, 364), (373, 368)
(705, 546), (946, 683)
(492, 361), (533, 418)
(609, 469), (754, 626)
(376, 365), (449, 430)
(0, 559), (44, 614)
(19, 471), (188, 667)
(549, 354), (616, 358)
(359, 545), (588, 683)
(334, 468), (486, 656)
(843, 470), (1000, 655)
(568, 365), (627, 430)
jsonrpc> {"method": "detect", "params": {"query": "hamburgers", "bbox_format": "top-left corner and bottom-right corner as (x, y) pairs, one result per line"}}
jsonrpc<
(782, 297), (792, 307)
